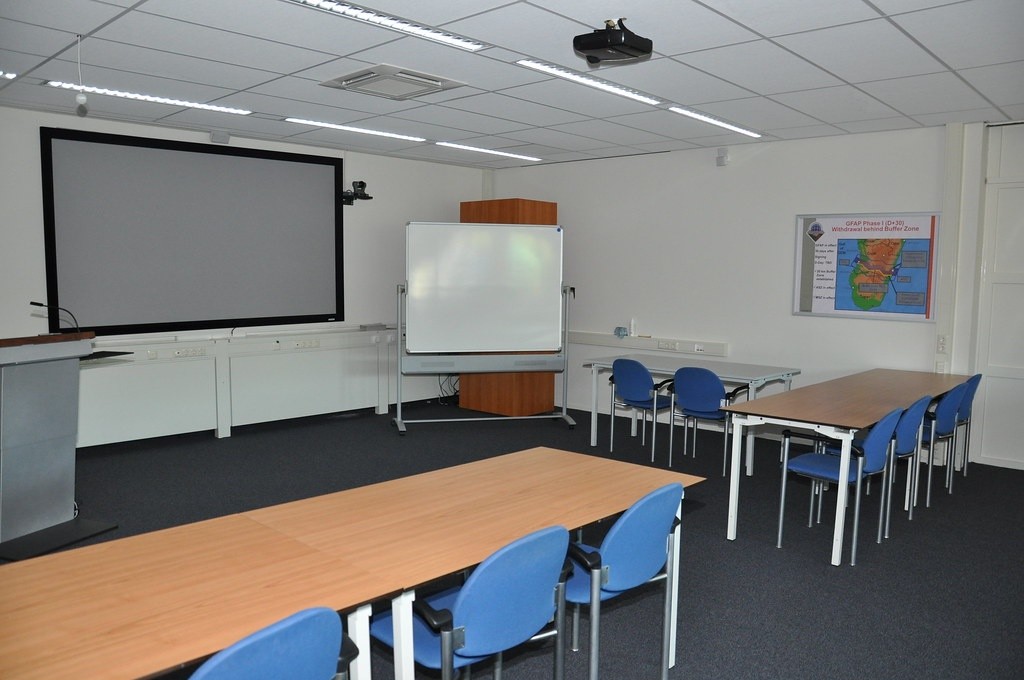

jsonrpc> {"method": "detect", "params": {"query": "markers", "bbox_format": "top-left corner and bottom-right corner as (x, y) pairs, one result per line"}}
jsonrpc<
(327, 317), (340, 321)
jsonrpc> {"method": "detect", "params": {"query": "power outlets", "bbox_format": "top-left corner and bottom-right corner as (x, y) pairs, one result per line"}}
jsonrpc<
(658, 340), (679, 350)
(148, 350), (158, 360)
(293, 338), (321, 349)
(273, 341), (280, 351)
(174, 346), (207, 359)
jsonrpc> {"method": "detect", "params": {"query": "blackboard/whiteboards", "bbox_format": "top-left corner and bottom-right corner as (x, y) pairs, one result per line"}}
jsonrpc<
(404, 222), (564, 354)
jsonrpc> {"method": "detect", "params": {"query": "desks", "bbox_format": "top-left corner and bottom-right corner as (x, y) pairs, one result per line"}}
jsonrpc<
(245, 445), (709, 680)
(715, 367), (973, 568)
(584, 353), (801, 478)
(0, 513), (404, 680)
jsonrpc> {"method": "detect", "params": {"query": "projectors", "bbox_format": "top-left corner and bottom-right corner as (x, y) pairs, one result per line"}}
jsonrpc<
(574, 29), (653, 64)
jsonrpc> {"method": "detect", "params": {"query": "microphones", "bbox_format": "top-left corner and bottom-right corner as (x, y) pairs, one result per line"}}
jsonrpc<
(31, 301), (80, 333)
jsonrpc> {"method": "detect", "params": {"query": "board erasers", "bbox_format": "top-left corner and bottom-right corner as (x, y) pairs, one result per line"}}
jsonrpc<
(360, 323), (387, 332)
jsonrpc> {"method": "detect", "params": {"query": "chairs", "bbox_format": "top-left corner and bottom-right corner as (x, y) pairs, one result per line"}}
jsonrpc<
(667, 366), (748, 478)
(608, 358), (688, 463)
(183, 606), (361, 680)
(773, 406), (904, 567)
(817, 394), (932, 542)
(494, 483), (684, 680)
(866, 382), (969, 510)
(929, 373), (983, 491)
(368, 525), (574, 680)
